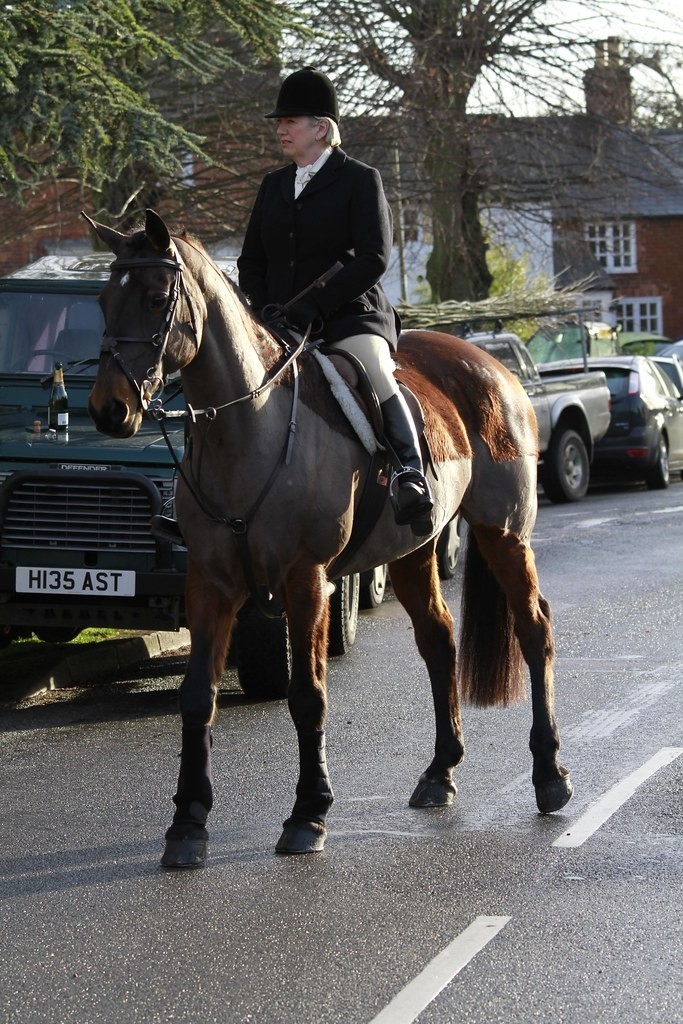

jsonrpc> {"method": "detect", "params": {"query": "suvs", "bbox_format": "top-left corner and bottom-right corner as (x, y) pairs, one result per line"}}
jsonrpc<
(0, 252), (362, 702)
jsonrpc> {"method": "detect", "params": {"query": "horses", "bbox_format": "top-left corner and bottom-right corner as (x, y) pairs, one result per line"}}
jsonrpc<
(84, 211), (572, 869)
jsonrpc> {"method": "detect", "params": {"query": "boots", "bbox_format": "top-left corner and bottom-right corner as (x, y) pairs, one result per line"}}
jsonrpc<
(380, 395), (433, 536)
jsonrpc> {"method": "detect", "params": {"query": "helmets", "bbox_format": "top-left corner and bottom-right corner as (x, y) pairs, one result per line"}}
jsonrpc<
(263, 66), (340, 126)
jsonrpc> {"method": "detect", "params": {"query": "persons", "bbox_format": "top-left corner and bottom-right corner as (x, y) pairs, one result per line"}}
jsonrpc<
(237, 66), (434, 537)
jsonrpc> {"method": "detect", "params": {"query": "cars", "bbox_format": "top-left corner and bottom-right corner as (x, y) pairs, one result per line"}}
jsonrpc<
(534, 340), (683, 492)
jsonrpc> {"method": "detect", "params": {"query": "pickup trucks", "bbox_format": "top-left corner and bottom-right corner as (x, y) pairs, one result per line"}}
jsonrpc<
(429, 319), (613, 503)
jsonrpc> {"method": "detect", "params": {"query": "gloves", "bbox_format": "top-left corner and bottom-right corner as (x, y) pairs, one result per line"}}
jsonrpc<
(253, 305), (278, 324)
(284, 294), (322, 327)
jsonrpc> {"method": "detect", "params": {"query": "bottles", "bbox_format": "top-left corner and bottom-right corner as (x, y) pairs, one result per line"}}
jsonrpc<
(48, 361), (69, 431)
